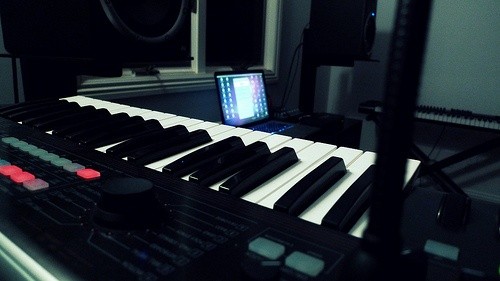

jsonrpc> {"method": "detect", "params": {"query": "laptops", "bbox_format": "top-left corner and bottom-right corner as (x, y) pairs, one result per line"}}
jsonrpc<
(214, 68), (297, 135)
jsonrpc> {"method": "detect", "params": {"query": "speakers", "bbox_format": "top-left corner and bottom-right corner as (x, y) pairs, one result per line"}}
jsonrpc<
(301, 0), (377, 67)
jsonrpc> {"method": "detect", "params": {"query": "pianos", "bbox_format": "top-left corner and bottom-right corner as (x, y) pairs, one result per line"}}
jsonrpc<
(356, 99), (500, 136)
(1, 92), (500, 281)
